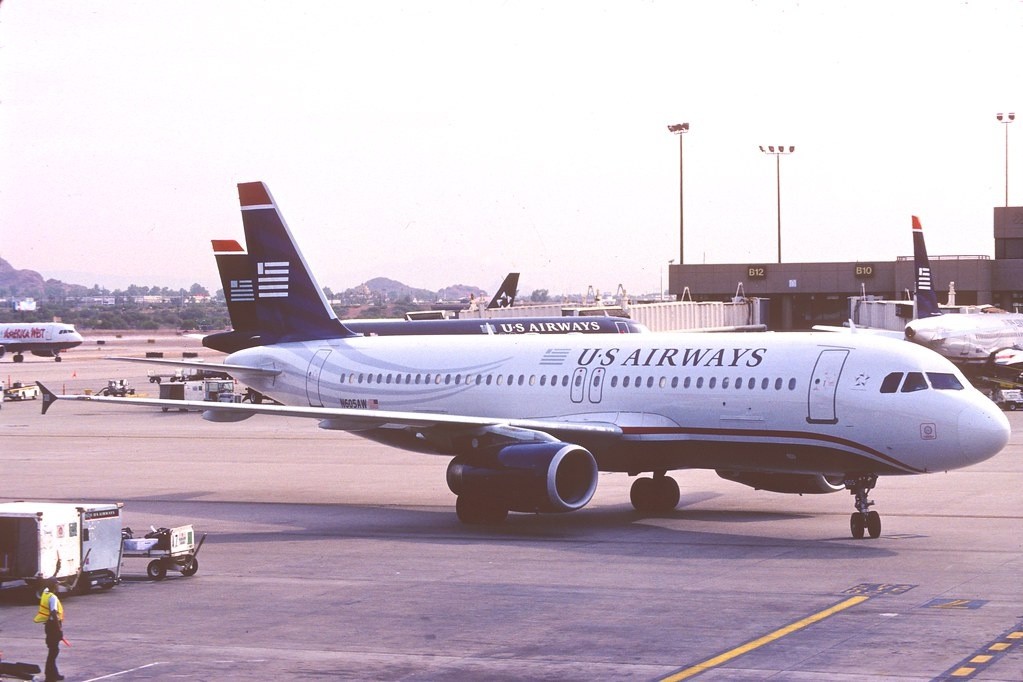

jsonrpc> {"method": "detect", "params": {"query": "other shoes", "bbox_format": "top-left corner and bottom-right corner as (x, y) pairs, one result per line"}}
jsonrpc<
(45, 675), (64, 682)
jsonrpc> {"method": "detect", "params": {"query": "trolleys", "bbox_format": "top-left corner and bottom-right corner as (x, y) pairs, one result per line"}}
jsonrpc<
(123, 532), (207, 581)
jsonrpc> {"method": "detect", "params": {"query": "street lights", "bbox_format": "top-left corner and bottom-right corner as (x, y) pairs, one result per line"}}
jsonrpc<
(759, 145), (795, 263)
(668, 122), (688, 262)
(997, 112), (1016, 206)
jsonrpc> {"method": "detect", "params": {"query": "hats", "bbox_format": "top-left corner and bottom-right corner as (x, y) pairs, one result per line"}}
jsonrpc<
(47, 577), (61, 587)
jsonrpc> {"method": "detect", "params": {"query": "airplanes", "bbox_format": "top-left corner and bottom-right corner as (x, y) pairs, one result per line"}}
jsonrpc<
(812, 215), (1023, 378)
(0, 322), (83, 363)
(36, 180), (1012, 538)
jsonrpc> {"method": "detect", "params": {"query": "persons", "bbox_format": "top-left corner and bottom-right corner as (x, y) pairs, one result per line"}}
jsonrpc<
(33, 577), (65, 682)
